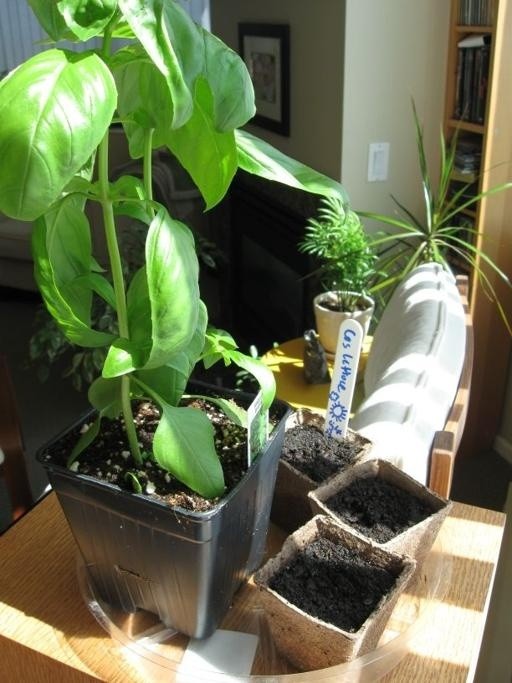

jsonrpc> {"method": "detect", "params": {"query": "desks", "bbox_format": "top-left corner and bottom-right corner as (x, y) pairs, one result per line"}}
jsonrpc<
(0, 485), (512, 682)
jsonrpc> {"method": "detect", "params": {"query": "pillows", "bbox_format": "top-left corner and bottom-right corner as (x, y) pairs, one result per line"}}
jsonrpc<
(348, 261), (467, 486)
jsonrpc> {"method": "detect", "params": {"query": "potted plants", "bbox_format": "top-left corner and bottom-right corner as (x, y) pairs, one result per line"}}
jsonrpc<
(0, 0), (352, 640)
(297, 195), (386, 362)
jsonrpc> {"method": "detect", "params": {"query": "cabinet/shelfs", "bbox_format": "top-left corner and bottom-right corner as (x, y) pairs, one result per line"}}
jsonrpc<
(443, 0), (512, 455)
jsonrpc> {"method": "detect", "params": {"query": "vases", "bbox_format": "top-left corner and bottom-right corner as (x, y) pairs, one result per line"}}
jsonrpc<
(245, 511), (417, 670)
(270, 405), (374, 535)
(306, 456), (454, 587)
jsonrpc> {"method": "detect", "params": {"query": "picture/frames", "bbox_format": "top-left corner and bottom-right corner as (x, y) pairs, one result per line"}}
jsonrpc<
(237, 19), (291, 138)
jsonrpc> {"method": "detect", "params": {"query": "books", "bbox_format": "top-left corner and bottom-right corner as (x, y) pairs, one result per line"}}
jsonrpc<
(454, 33), (494, 126)
(446, 132), (480, 208)
(460, 1), (499, 26)
(443, 207), (477, 278)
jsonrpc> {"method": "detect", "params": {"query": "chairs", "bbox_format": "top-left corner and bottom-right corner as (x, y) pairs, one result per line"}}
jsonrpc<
(428, 273), (475, 500)
